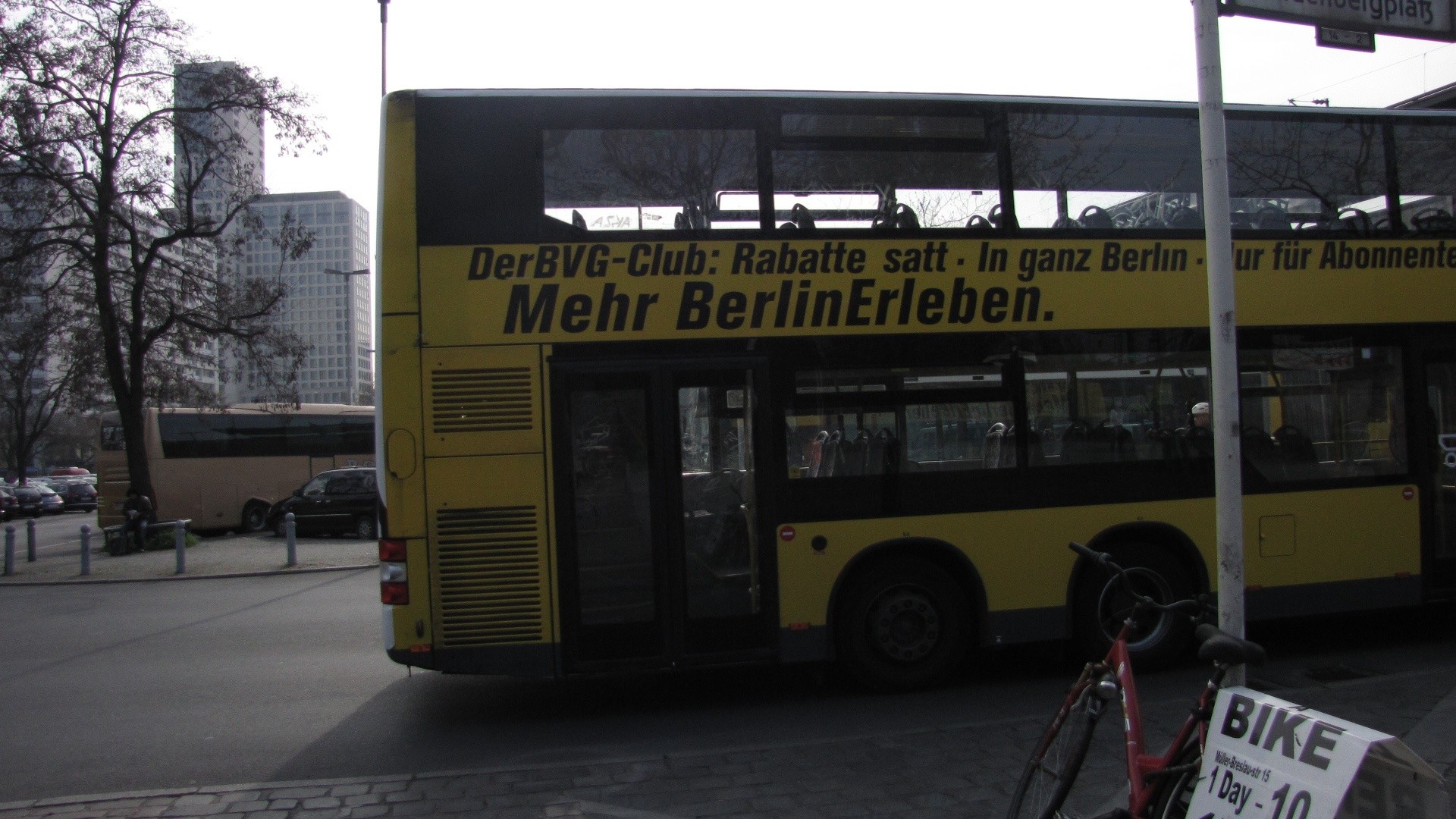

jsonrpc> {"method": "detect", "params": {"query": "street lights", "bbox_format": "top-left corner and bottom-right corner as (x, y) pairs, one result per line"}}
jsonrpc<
(322, 267), (371, 405)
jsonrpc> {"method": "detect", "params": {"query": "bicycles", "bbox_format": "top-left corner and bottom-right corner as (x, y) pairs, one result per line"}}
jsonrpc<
(999, 538), (1263, 819)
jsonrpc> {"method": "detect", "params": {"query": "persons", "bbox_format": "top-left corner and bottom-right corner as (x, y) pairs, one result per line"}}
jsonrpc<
(1191, 403), (1209, 426)
(120, 488), (152, 552)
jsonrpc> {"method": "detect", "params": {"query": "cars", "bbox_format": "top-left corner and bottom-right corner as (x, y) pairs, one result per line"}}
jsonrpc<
(94, 399), (381, 535)
(265, 467), (379, 540)
(0, 466), (98, 522)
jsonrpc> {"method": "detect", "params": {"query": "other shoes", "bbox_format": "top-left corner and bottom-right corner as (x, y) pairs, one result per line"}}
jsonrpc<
(140, 548), (146, 553)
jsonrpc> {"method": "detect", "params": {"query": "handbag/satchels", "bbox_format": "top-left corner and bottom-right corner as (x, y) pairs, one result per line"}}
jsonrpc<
(110, 537), (132, 556)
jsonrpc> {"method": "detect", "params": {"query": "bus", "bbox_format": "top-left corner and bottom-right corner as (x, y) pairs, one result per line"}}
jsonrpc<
(365, 84), (1456, 704)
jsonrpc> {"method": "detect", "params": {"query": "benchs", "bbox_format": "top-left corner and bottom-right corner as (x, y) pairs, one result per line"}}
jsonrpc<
(103, 518), (192, 549)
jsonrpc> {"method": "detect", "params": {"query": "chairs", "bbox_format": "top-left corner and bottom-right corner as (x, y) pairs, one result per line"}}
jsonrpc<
(573, 207), (1456, 230)
(805, 423), (1328, 478)
